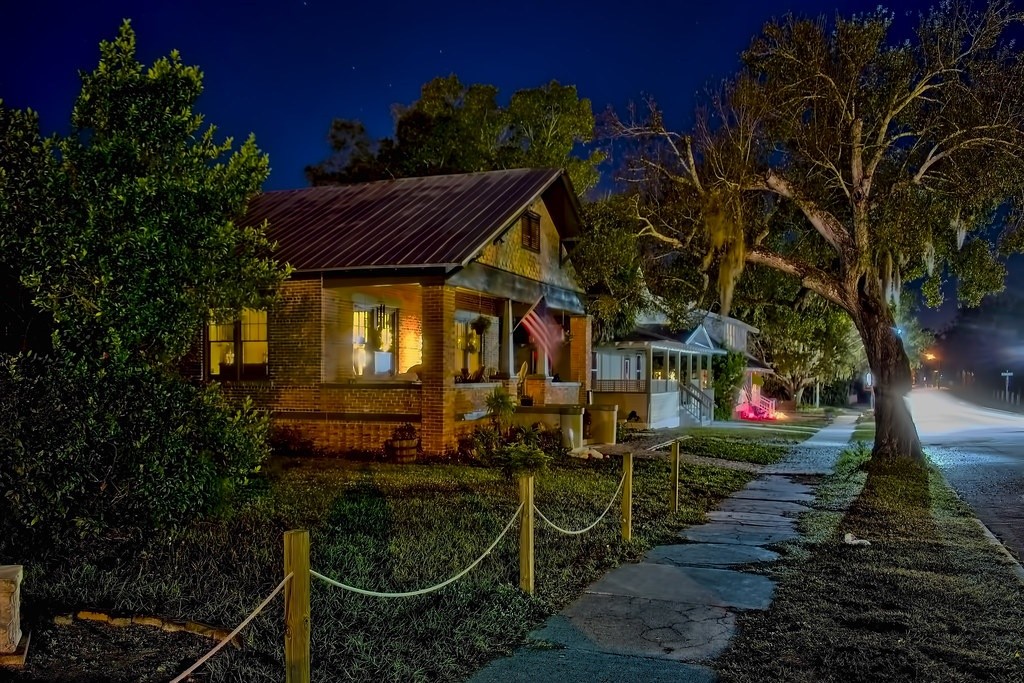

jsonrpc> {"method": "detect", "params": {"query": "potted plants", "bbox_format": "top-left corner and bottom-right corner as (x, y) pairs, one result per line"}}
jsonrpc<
(388, 423), (423, 462)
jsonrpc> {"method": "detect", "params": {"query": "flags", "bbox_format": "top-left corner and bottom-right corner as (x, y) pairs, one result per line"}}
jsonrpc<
(521, 295), (565, 366)
